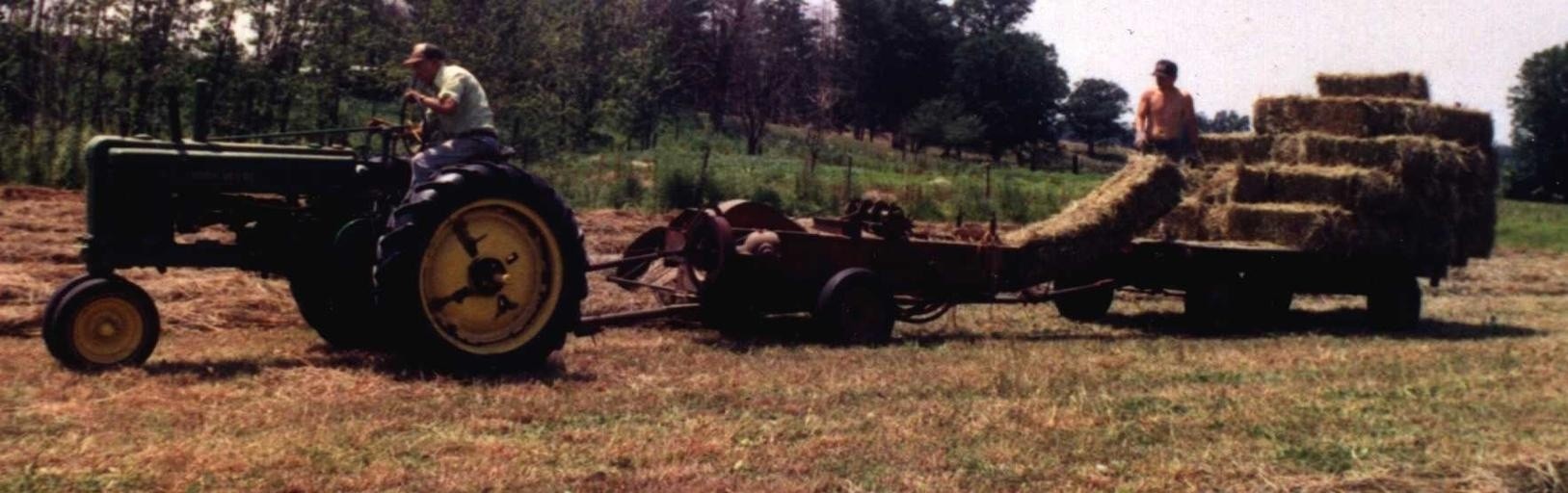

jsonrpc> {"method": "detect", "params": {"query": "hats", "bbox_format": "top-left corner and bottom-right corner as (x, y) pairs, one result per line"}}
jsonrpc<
(404, 43), (444, 65)
(1152, 61), (1176, 76)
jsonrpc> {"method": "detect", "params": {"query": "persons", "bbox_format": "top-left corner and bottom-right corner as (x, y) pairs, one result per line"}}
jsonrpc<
(399, 41), (502, 201)
(1134, 58), (1198, 175)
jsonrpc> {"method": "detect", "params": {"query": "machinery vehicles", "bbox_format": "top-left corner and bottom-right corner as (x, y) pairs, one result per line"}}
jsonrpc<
(30, 78), (598, 394)
